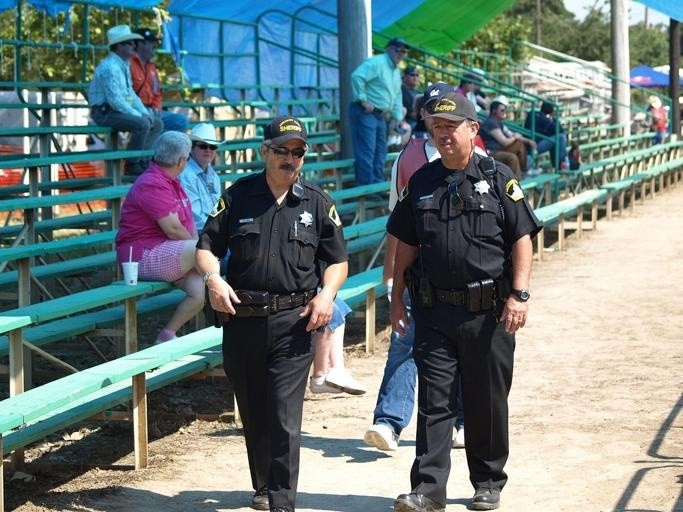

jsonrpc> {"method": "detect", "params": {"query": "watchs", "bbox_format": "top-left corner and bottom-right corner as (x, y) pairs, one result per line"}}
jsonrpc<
(200, 268), (220, 284)
(509, 283), (531, 304)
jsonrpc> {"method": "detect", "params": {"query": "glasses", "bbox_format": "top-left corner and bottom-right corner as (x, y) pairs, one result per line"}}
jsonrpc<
(448, 180), (464, 210)
(197, 144), (217, 150)
(264, 144), (306, 159)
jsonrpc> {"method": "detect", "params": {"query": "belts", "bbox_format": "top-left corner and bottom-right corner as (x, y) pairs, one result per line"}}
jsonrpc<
(269, 290), (315, 318)
(434, 290), (465, 306)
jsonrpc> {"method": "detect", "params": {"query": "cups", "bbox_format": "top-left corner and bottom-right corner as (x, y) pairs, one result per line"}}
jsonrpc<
(121, 262), (139, 285)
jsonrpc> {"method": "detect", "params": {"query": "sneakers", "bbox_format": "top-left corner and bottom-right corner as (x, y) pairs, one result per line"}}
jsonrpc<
(364, 425), (398, 451)
(454, 429), (465, 448)
(124, 162), (148, 176)
(327, 366), (365, 395)
(156, 328), (177, 343)
(527, 168), (543, 177)
(310, 376), (341, 394)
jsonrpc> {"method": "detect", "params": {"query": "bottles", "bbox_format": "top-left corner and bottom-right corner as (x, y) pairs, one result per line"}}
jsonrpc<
(565, 155), (569, 170)
(389, 123), (407, 135)
(527, 155), (533, 172)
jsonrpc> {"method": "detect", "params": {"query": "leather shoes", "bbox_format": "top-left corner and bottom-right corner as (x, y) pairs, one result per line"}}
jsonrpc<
(471, 486), (500, 509)
(253, 487), (270, 510)
(395, 493), (444, 512)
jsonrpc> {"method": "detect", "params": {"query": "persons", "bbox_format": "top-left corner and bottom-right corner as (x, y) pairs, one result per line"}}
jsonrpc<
(196, 113), (349, 512)
(361, 87), (500, 452)
(390, 93), (534, 511)
(130, 24), (191, 138)
(645, 97), (671, 144)
(307, 283), (369, 396)
(117, 132), (211, 348)
(90, 25), (157, 182)
(347, 35), (571, 190)
(177, 121), (241, 271)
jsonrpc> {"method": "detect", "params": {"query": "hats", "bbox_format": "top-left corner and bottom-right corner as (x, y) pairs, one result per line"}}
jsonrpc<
(264, 117), (311, 148)
(494, 95), (509, 105)
(648, 96), (661, 109)
(384, 37), (408, 49)
(420, 93), (478, 123)
(403, 66), (420, 75)
(190, 124), (217, 146)
(135, 28), (160, 41)
(470, 68), (488, 84)
(421, 83), (453, 113)
(107, 25), (144, 46)
